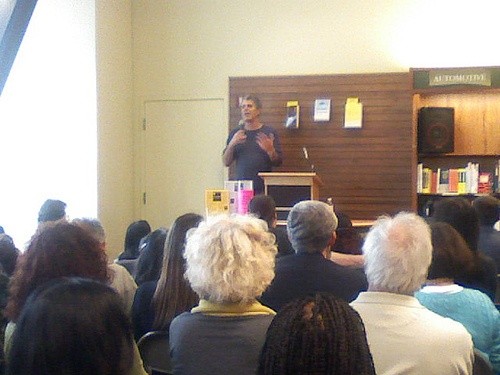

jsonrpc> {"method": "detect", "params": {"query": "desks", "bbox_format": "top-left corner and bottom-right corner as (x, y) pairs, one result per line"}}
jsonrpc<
(275, 219), (379, 235)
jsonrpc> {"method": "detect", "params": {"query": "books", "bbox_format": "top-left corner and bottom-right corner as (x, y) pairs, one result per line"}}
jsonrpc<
(205, 180), (254, 215)
(416, 160), (500, 196)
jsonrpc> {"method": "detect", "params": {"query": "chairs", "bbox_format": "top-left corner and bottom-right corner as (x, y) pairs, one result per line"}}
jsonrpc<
(473, 351), (493, 375)
(136, 329), (173, 375)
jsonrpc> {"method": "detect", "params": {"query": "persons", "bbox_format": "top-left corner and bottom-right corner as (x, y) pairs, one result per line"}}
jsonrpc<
(331, 215), (365, 255)
(256, 200), (369, 314)
(114, 219), (151, 263)
(472, 196), (500, 275)
(6, 216), (149, 375)
(348, 210), (474, 374)
(132, 228), (169, 286)
(0, 234), (20, 324)
(247, 194), (365, 269)
(0, 225), (5, 233)
(72, 218), (139, 317)
(37, 199), (67, 222)
(430, 196), (496, 272)
(169, 210), (277, 375)
(4, 275), (135, 375)
(222, 94), (284, 195)
(130, 212), (205, 343)
(256, 292), (376, 374)
(413, 222), (500, 374)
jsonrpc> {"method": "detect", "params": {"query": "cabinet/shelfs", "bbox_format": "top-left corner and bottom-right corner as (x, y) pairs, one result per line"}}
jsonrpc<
(414, 89), (500, 198)
